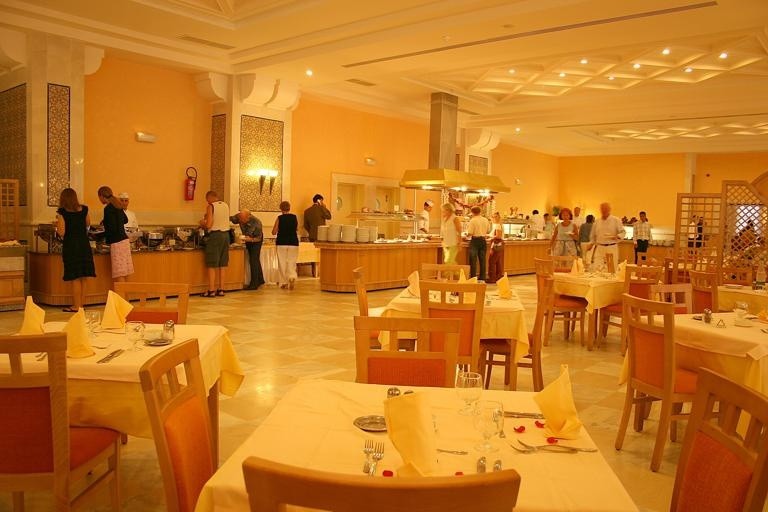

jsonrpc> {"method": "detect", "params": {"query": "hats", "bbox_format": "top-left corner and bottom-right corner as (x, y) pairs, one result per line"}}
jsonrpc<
(117, 192), (128, 199)
(425, 199), (434, 207)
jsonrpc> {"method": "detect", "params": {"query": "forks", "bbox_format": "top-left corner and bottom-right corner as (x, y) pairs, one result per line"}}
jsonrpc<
(492, 409), (505, 437)
(363, 439), (373, 473)
(368, 442), (384, 477)
(511, 440), (599, 454)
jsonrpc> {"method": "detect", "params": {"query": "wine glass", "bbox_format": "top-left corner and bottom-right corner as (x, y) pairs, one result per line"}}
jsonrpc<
(124, 321), (145, 352)
(473, 398), (504, 453)
(456, 371), (483, 415)
(83, 310), (101, 344)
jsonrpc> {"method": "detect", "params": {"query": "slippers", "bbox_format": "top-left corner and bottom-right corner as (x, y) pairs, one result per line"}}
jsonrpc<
(63, 307), (85, 312)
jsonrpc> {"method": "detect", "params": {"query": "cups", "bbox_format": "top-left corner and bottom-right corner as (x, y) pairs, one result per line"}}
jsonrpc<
(735, 303), (748, 321)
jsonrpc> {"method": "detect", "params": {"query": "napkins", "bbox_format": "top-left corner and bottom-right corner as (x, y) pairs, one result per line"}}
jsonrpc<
(533, 363), (584, 440)
(61, 307), (97, 359)
(407, 270), (420, 297)
(19, 295), (47, 334)
(618, 259), (627, 272)
(101, 288), (133, 327)
(494, 272), (514, 300)
(383, 389), (441, 475)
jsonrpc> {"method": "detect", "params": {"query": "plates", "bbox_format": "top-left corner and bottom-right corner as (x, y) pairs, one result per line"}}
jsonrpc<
(725, 283), (742, 289)
(145, 338), (172, 346)
(317, 224), (380, 243)
(354, 415), (388, 431)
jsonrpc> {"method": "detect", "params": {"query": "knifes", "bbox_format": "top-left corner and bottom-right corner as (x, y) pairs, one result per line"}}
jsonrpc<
(493, 459), (502, 472)
(476, 456), (486, 474)
(497, 410), (543, 419)
(98, 348), (124, 364)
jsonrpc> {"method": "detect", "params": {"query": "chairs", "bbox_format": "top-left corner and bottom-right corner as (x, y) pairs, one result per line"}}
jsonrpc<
(353, 317), (457, 390)
(418, 281), (489, 387)
(420, 264), (470, 282)
(1, 331), (129, 511)
(353, 265), (416, 352)
(532, 253), (766, 352)
(242, 455), (521, 512)
(114, 281), (189, 326)
(139, 338), (220, 511)
(614, 291), (725, 473)
(481, 272), (554, 393)
(670, 365), (768, 511)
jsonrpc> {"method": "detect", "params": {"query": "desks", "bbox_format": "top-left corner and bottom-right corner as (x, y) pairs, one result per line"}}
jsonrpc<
(0, 239), (30, 312)
(377, 276), (530, 393)
(311, 238), (551, 295)
(615, 239), (674, 266)
(192, 375), (641, 511)
(1, 321), (244, 476)
(28, 252), (245, 305)
(632, 311), (767, 399)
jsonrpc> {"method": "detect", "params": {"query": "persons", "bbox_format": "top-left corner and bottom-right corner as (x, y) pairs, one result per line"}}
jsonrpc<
(440, 203), (463, 281)
(465, 206), (491, 283)
(572, 207), (585, 227)
(95, 185), (134, 282)
(737, 219), (758, 237)
(417, 199), (434, 234)
(687, 215), (700, 247)
(302, 193), (332, 277)
(577, 214), (595, 242)
(697, 216), (707, 247)
(632, 211), (653, 264)
(199, 190), (231, 298)
(585, 202), (627, 265)
(271, 200), (300, 290)
(56, 188), (97, 313)
(528, 209), (544, 232)
(117, 192), (139, 230)
(542, 213), (555, 234)
(486, 212), (505, 283)
(549, 207), (580, 272)
(228, 208), (266, 290)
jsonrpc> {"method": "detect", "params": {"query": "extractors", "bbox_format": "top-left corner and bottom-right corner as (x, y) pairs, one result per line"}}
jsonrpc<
(398, 93), (512, 193)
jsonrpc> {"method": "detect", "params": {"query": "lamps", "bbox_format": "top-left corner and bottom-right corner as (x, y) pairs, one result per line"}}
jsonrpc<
(258, 175), (277, 196)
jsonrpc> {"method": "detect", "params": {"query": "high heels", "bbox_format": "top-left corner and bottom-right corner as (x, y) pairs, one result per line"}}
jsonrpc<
(280, 284), (289, 289)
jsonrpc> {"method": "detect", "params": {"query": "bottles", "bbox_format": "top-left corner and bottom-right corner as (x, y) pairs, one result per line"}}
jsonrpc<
(703, 308), (712, 323)
(162, 320), (174, 338)
(388, 388), (401, 399)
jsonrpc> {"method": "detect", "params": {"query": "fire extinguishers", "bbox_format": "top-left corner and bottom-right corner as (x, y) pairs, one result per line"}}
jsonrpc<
(185, 167), (197, 200)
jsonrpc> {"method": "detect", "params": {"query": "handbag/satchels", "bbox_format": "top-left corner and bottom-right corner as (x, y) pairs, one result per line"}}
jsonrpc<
(491, 241), (503, 251)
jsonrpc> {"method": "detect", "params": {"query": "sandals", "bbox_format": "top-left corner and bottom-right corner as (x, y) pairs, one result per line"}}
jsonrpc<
(202, 290), (225, 297)
